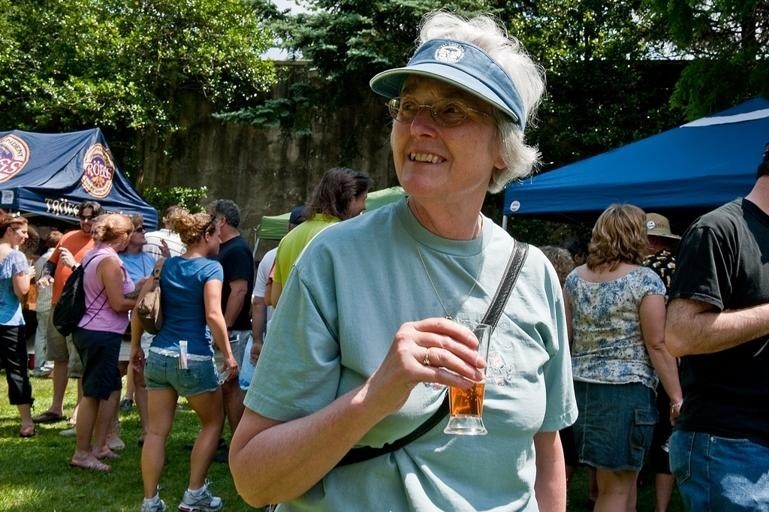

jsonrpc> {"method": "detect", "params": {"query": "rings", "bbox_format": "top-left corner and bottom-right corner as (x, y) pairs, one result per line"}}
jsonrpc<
(423, 348), (430, 365)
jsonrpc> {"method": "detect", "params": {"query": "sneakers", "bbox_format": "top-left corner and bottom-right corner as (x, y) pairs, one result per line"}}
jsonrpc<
(70, 433), (124, 470)
(32, 412), (62, 422)
(36, 366), (52, 377)
(142, 498), (166, 512)
(177, 479), (222, 512)
(120, 399), (132, 408)
(59, 430), (75, 436)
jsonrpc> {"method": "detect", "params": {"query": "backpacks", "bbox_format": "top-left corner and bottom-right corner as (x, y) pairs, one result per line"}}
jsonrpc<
(53, 253), (108, 336)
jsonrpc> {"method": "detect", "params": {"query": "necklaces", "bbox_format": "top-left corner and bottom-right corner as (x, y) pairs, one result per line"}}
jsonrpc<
(405, 197), (485, 319)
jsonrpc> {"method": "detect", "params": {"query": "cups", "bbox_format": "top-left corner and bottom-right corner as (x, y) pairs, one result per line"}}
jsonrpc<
(442, 320), (491, 437)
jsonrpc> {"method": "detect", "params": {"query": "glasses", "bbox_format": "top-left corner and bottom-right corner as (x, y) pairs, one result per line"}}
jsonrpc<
(385, 97), (490, 128)
(80, 215), (91, 220)
(134, 227), (144, 232)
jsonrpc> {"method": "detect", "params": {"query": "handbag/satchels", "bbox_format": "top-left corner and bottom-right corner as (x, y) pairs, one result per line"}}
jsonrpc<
(138, 278), (164, 334)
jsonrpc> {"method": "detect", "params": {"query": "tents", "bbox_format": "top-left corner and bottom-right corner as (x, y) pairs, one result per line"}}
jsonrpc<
(254, 186), (408, 261)
(504, 102), (768, 235)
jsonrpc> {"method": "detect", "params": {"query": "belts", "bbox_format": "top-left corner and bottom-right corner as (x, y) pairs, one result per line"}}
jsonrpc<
(152, 347), (212, 361)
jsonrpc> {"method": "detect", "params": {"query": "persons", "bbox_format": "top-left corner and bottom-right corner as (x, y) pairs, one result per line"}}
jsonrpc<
(229, 13), (578, 512)
(663, 135), (769, 512)
(540, 202), (686, 512)
(0, 167), (372, 512)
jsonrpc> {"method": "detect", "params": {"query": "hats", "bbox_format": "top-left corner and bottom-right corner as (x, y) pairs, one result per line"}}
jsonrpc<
(646, 213), (682, 239)
(369, 39), (526, 133)
